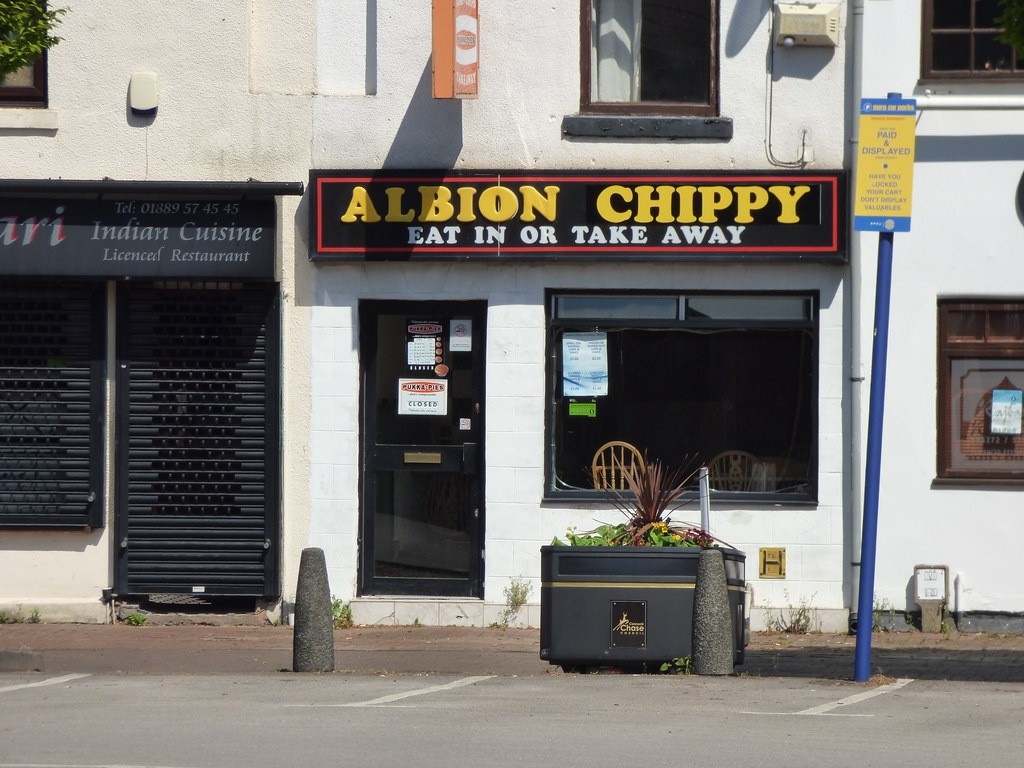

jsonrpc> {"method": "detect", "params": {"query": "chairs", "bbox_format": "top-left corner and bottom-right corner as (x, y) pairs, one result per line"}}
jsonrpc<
(592, 441), (647, 490)
(708, 451), (766, 491)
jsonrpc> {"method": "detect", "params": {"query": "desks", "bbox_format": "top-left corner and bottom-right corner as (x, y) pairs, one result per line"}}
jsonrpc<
(694, 464), (808, 482)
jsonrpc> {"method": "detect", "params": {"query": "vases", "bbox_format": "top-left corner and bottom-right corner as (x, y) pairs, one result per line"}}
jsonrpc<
(539, 545), (748, 672)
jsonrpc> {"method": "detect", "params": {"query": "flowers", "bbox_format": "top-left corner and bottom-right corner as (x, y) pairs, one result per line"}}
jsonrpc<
(549, 448), (742, 552)
